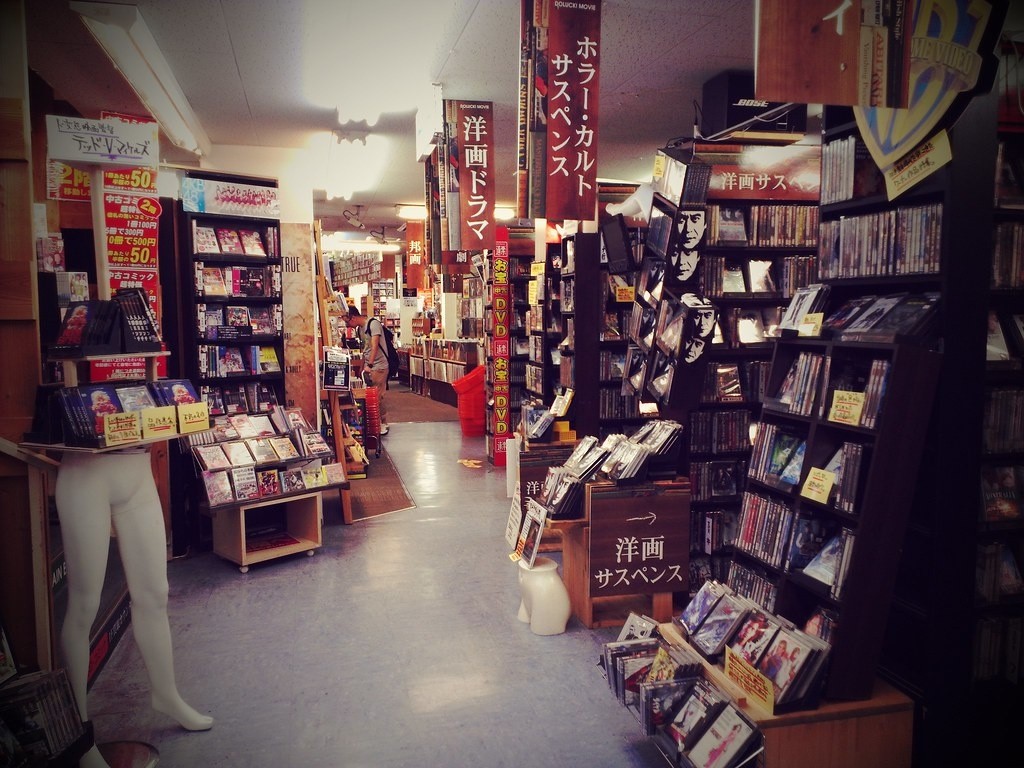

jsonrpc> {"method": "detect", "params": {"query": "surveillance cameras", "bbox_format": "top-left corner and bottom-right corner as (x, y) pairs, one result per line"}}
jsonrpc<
(348, 218), (363, 228)
(374, 235), (386, 244)
(397, 223), (407, 233)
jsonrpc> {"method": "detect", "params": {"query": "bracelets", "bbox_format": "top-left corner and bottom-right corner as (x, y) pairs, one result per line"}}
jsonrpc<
(367, 364), (374, 368)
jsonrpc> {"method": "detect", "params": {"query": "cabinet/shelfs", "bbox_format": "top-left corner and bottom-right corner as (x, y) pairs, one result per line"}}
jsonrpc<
(410, 106), (1023, 768)
(17, 351), (217, 454)
(161, 165), (397, 574)
(0, 667), (95, 768)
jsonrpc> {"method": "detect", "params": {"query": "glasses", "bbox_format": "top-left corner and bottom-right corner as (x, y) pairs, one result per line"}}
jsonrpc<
(345, 318), (352, 324)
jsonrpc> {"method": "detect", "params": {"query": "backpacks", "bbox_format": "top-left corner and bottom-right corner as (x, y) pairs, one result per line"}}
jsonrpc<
(358, 318), (400, 377)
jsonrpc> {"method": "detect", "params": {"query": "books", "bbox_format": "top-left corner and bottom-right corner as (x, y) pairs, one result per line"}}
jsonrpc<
(511, 238), (574, 439)
(187, 219), (345, 507)
(408, 276), (483, 385)
(37, 236), (202, 445)
(602, 139), (943, 768)
(535, 148), (819, 596)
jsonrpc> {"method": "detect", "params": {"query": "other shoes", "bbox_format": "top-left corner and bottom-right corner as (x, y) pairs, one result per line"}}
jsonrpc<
(381, 425), (389, 434)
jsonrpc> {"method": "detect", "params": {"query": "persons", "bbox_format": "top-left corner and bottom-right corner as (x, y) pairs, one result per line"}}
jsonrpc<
(775, 647), (800, 691)
(70, 273), (88, 301)
(822, 539), (841, 567)
(680, 293), (712, 308)
(342, 306), (390, 435)
(796, 523), (815, 556)
(765, 640), (785, 689)
(551, 479), (571, 505)
(56, 442), (215, 767)
(219, 351), (239, 377)
(261, 362), (270, 372)
(675, 700), (699, 734)
(677, 211), (707, 249)
(670, 251), (701, 281)
(44, 253), (54, 272)
(694, 309), (716, 337)
(541, 470), (555, 505)
(731, 619), (760, 654)
(684, 336), (705, 363)
(54, 253), (63, 271)
(703, 724), (742, 768)
